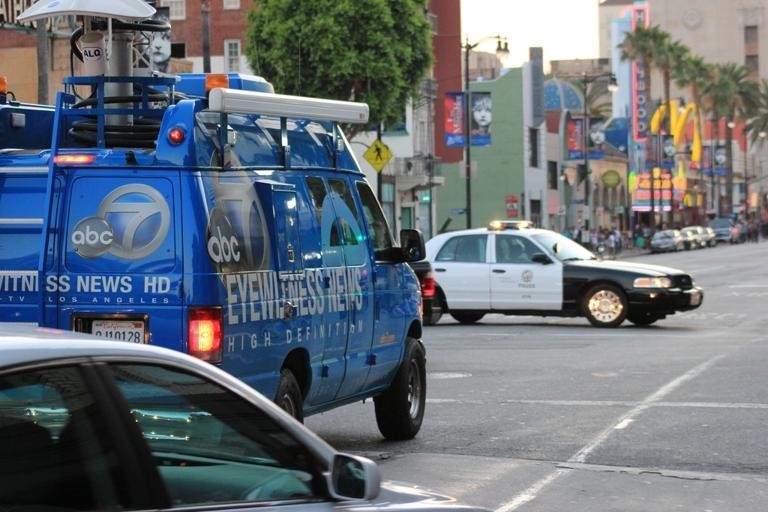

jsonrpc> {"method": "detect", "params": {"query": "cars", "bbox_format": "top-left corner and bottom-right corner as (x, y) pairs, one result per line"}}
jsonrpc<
(649, 216), (740, 252)
(417, 218), (703, 328)
(0, 320), (491, 511)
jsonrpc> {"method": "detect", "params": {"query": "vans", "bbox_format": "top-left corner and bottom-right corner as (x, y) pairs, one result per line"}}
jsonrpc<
(0, 0), (427, 441)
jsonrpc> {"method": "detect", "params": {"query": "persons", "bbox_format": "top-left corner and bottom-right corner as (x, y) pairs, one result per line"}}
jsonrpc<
(564, 223), (645, 259)
(728, 215), (758, 246)
(142, 17), (170, 64)
(590, 123), (604, 146)
(473, 97), (492, 132)
(715, 147), (726, 164)
(663, 138), (675, 157)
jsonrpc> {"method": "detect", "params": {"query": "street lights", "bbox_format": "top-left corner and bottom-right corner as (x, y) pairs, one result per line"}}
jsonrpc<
(580, 72), (619, 253)
(711, 113), (735, 214)
(742, 119), (767, 219)
(655, 98), (685, 230)
(458, 35), (510, 228)
